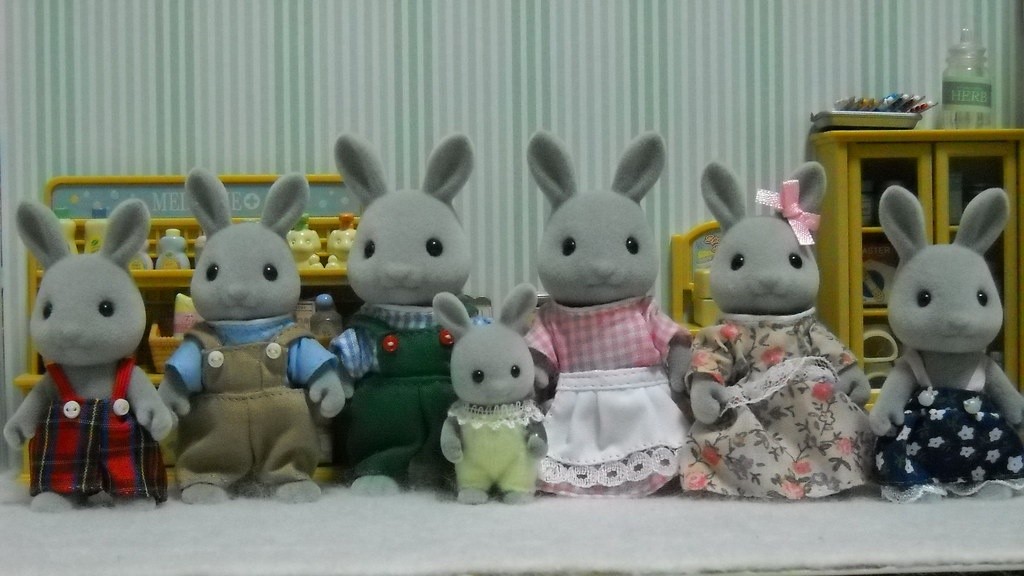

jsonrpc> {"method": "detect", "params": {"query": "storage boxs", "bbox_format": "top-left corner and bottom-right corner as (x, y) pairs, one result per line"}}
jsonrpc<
(147, 318), (188, 374)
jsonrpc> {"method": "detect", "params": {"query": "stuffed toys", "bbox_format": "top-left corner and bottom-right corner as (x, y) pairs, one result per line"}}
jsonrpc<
(867, 185), (1023, 504)
(328, 134), (496, 499)
(158, 167), (343, 505)
(3, 199), (172, 515)
(677, 162), (875, 499)
(524, 131), (695, 497)
(431, 283), (548, 506)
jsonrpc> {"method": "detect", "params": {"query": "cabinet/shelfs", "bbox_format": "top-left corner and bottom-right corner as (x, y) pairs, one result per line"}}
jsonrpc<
(16, 174), (364, 482)
(812, 128), (1022, 405)
